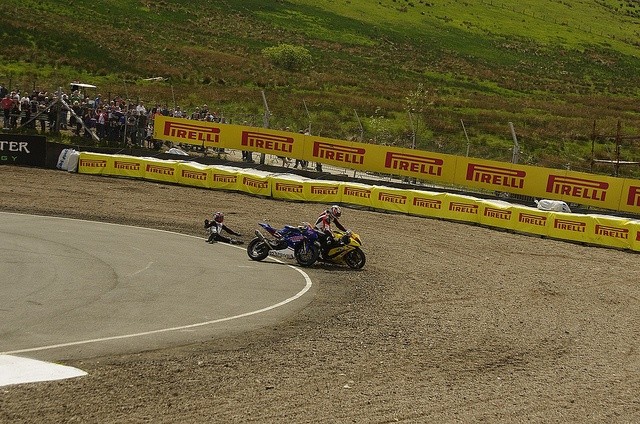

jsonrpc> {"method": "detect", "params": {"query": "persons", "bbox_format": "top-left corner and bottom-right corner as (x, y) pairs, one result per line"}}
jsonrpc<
(203, 212), (244, 245)
(173, 104), (227, 154)
(368, 138), (374, 145)
(402, 144), (417, 184)
(84, 93), (174, 150)
(0, 81), (84, 135)
(391, 142), (397, 147)
(241, 121), (248, 159)
(312, 204), (347, 259)
(287, 157), (292, 166)
(278, 125), (290, 168)
(293, 130), (305, 168)
(304, 129), (311, 166)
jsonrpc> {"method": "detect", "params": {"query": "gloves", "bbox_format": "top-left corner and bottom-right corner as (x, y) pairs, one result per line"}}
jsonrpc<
(204, 219), (209, 225)
(237, 232), (242, 237)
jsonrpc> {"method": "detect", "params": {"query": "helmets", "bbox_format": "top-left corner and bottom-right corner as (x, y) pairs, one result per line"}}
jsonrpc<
(331, 205), (342, 217)
(214, 212), (224, 222)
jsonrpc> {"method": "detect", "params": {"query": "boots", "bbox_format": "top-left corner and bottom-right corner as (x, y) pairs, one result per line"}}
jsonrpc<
(321, 252), (332, 260)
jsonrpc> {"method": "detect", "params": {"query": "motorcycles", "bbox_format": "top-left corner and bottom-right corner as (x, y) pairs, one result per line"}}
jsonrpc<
(247, 222), (321, 266)
(307, 226), (366, 269)
